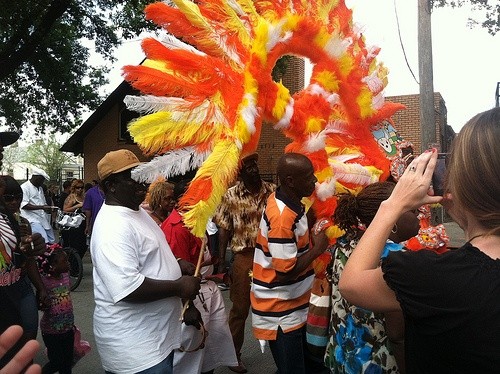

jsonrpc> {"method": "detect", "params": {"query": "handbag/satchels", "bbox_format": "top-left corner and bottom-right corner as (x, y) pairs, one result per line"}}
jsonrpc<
(44, 324), (91, 368)
(306, 273), (332, 347)
(55, 208), (87, 231)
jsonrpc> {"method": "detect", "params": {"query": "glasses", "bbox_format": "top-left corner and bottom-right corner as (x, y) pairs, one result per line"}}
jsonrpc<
(75, 185), (84, 189)
(2, 192), (23, 202)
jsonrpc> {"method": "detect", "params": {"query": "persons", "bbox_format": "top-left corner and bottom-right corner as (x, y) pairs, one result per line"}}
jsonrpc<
(90, 149), (202, 374)
(160, 179), (239, 374)
(145, 180), (175, 226)
(339, 106), (500, 374)
(250, 152), (330, 374)
(217, 145), (278, 370)
(324, 181), (422, 374)
(0, 131), (106, 374)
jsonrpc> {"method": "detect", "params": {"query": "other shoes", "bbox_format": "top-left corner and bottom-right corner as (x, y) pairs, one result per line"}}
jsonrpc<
(228, 361), (247, 374)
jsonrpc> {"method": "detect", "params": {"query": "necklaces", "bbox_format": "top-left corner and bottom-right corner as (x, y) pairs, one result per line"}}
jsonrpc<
(467, 229), (491, 244)
(75, 194), (85, 204)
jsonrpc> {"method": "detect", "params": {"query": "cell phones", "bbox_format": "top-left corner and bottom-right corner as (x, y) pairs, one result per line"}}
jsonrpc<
(430, 152), (449, 196)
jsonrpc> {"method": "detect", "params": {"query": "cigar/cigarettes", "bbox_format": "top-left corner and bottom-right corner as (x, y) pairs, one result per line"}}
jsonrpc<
(29, 236), (35, 250)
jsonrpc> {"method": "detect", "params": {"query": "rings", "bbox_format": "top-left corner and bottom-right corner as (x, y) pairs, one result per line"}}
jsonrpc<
(409, 166), (416, 172)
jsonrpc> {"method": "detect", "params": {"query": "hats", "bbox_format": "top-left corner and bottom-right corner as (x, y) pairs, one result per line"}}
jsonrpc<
(32, 169), (50, 182)
(236, 150), (259, 181)
(97, 149), (150, 181)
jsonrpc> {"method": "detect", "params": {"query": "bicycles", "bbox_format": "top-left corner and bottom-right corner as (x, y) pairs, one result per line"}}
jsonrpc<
(48, 206), (87, 294)
